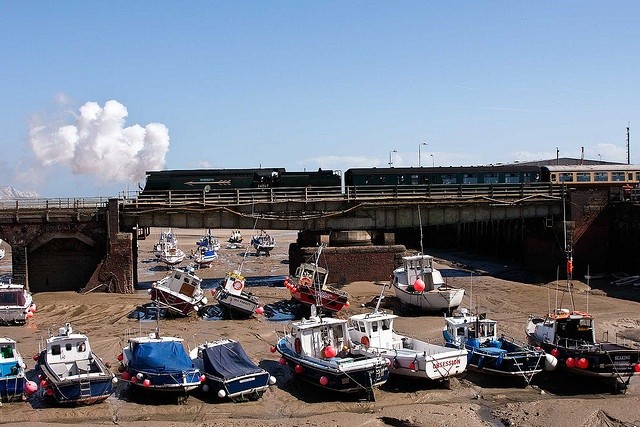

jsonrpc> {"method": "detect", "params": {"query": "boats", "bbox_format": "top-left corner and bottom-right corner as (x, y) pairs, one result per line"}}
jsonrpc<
(269, 305), (391, 395)
(251, 226), (276, 258)
(525, 234), (640, 381)
(443, 274), (558, 387)
(211, 213), (259, 320)
(31, 323), (120, 406)
(390, 204), (466, 310)
(158, 247), (186, 269)
(346, 311), (470, 383)
(145, 266), (209, 321)
(187, 334), (278, 402)
(0, 335), (38, 405)
(284, 241), (351, 316)
(153, 225), (179, 257)
(115, 306), (208, 399)
(191, 241), (218, 270)
(230, 229), (242, 243)
(199, 225), (221, 252)
(0, 274), (38, 328)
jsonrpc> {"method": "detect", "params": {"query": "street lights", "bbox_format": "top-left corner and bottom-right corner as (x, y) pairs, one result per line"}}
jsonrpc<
(389, 149), (400, 171)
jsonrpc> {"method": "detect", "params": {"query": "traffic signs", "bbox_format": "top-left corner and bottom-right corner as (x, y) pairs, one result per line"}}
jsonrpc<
(430, 154), (437, 170)
(417, 142), (429, 168)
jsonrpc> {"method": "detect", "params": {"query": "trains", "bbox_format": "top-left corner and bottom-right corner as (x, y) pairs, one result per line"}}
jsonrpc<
(137, 161), (636, 195)
